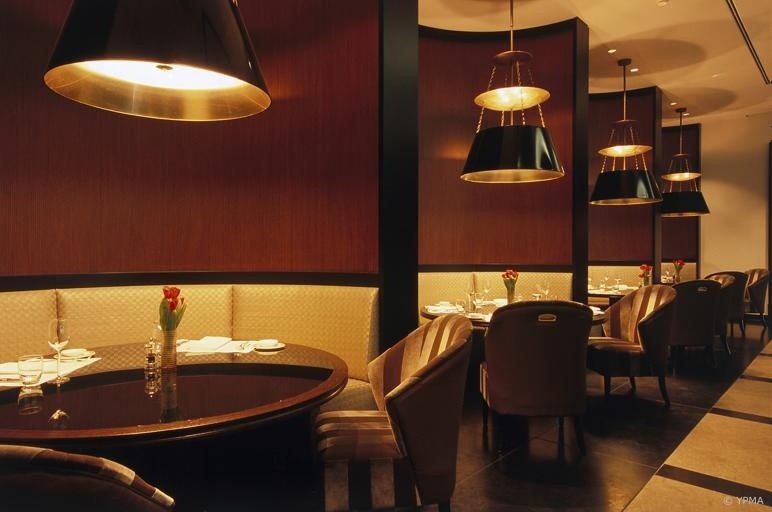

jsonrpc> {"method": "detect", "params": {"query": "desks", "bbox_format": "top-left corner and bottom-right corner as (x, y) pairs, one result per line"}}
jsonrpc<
(1, 442), (178, 512)
(311, 312), (474, 512)
(589, 275), (693, 306)
(1, 339), (350, 512)
(418, 297), (609, 453)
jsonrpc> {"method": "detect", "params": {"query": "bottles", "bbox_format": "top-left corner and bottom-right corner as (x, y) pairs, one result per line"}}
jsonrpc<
(146, 337), (163, 368)
(145, 369), (163, 397)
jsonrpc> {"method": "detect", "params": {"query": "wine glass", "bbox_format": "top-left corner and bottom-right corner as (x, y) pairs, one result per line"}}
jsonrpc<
(47, 385), (72, 430)
(47, 318), (72, 385)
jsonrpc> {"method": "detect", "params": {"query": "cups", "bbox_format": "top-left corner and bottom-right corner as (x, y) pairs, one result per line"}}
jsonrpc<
(18, 384), (45, 416)
(17, 354), (47, 384)
(528, 279), (558, 301)
(588, 271), (621, 295)
(456, 277), (492, 313)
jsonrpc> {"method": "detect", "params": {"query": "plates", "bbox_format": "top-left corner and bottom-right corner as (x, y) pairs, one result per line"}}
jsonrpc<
(62, 348), (87, 356)
(254, 342), (286, 351)
(258, 339), (280, 346)
(425, 296), (507, 324)
(53, 350), (96, 360)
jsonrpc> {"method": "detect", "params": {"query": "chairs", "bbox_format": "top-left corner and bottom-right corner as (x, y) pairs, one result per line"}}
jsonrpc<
(478, 300), (593, 472)
(667, 268), (769, 370)
(587, 283), (677, 411)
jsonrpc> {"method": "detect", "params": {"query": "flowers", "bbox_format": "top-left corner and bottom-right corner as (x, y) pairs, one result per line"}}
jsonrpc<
(673, 260), (685, 275)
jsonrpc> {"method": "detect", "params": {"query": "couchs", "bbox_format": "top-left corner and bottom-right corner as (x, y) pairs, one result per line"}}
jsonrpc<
(417, 264), (587, 418)
(586, 264), (699, 290)
(2, 284), (380, 412)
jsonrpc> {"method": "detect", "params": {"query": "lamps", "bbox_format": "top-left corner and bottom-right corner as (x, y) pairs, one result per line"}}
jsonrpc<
(41, 0), (272, 123)
(659, 108), (710, 217)
(589, 59), (663, 206)
(459, 0), (565, 184)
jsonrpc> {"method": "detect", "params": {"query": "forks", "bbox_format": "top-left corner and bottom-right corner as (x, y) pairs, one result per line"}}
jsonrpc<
(235, 341), (251, 351)
